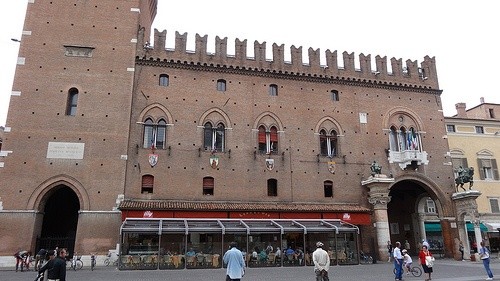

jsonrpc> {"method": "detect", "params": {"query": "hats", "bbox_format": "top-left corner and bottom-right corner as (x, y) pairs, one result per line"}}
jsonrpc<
(229, 242), (237, 246)
(316, 242), (324, 247)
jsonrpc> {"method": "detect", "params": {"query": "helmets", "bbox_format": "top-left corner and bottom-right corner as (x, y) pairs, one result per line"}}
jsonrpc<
(402, 249), (407, 252)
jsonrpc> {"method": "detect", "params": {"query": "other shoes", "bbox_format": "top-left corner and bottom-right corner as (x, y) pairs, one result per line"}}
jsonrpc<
(395, 278), (403, 281)
(487, 277), (493, 280)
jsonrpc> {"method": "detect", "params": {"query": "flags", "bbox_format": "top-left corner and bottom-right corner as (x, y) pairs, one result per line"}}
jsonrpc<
(412, 128), (419, 150)
(405, 129), (413, 149)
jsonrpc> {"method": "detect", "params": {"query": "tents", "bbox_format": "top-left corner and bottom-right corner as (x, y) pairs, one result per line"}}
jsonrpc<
(118, 218), (362, 269)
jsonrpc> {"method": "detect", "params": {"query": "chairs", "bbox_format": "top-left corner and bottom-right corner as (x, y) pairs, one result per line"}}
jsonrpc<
(124, 248), (347, 268)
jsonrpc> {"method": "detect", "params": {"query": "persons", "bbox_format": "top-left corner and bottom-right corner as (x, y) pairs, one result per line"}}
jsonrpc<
(223, 241), (246, 281)
(458, 164), (467, 186)
(39, 249), (67, 281)
(370, 160), (382, 177)
(472, 239), (480, 254)
(236, 241), (310, 268)
(478, 240), (493, 281)
(458, 241), (468, 262)
(312, 241), (330, 281)
(392, 242), (405, 280)
(184, 247), (196, 268)
(401, 248), (414, 276)
(420, 245), (435, 280)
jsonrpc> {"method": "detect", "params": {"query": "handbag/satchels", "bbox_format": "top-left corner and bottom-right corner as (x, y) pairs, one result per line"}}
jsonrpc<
(480, 252), (489, 259)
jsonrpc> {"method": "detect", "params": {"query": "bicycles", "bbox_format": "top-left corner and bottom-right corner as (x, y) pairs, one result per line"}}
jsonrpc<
(105, 253), (119, 266)
(90, 253), (96, 271)
(393, 262), (422, 277)
(71, 252), (84, 271)
(360, 250), (374, 265)
